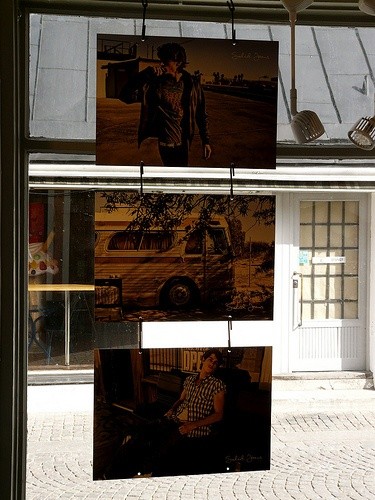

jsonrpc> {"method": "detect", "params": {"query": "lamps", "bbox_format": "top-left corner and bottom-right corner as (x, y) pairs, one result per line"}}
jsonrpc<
(347, 116), (375, 153)
(281, 0), (327, 145)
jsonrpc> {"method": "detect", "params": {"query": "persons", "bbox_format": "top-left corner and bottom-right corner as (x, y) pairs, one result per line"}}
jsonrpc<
(116, 42), (211, 167)
(96, 348), (229, 479)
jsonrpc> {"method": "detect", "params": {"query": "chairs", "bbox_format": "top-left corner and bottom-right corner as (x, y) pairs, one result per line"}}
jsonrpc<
(43, 298), (78, 367)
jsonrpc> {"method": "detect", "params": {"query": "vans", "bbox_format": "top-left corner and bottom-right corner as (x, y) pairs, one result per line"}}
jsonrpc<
(94, 212), (237, 315)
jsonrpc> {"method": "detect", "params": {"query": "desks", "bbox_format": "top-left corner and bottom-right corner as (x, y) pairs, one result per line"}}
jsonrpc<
(28, 304), (59, 365)
